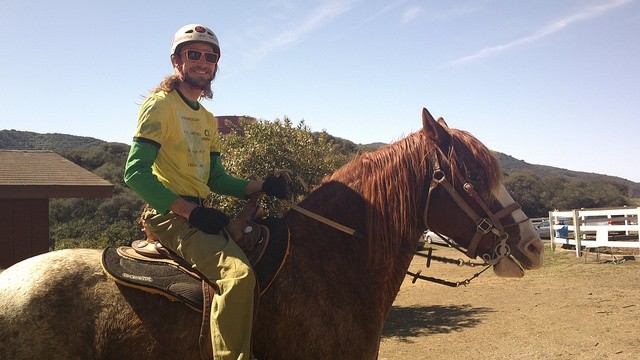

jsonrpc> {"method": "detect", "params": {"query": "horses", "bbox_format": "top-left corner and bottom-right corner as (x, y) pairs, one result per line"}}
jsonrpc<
(1, 104), (545, 360)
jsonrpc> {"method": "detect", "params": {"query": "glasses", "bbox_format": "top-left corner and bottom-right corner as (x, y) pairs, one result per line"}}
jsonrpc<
(178, 49), (218, 65)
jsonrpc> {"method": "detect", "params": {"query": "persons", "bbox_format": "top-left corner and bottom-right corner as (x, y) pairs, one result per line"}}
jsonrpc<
(122, 22), (290, 360)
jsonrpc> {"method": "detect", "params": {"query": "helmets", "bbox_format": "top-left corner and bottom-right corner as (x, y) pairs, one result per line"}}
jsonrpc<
(170, 24), (221, 55)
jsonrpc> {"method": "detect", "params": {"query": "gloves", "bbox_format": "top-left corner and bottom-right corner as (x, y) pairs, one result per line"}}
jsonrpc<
(263, 169), (293, 201)
(188, 206), (229, 234)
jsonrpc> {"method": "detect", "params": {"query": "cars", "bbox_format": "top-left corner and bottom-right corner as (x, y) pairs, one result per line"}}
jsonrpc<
(534, 220), (568, 239)
(424, 230), (456, 247)
